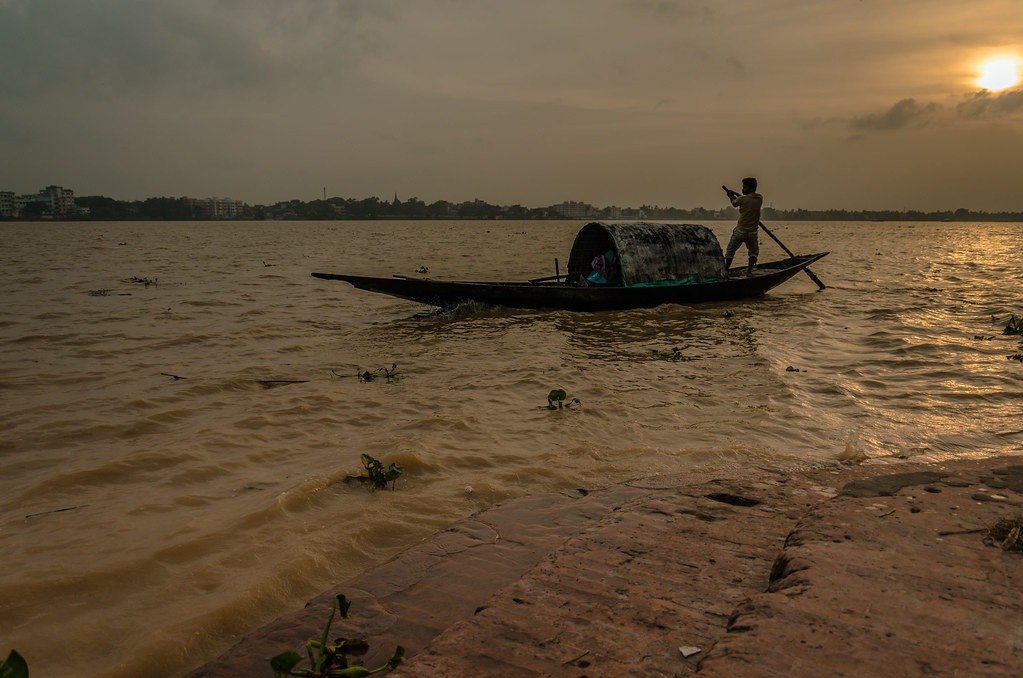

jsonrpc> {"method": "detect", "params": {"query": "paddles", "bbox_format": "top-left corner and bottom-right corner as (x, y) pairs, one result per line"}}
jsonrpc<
(722, 185), (826, 290)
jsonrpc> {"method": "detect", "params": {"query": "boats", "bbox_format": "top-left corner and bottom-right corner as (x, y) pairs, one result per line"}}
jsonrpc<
(311, 221), (833, 310)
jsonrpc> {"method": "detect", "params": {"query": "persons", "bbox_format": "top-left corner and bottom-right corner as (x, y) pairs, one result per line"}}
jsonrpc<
(724, 177), (763, 277)
